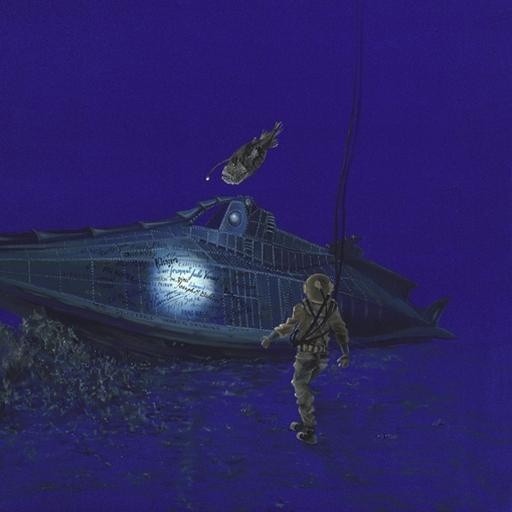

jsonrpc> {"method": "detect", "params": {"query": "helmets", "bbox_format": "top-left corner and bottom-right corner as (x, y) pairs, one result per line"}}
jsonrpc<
(303, 273), (334, 305)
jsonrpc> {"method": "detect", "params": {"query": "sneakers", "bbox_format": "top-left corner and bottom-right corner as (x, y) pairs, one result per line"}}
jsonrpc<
(289, 422), (304, 431)
(296, 431), (318, 445)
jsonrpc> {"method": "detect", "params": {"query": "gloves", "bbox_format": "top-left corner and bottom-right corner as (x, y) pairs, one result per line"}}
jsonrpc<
(336, 355), (351, 369)
(260, 336), (271, 350)
